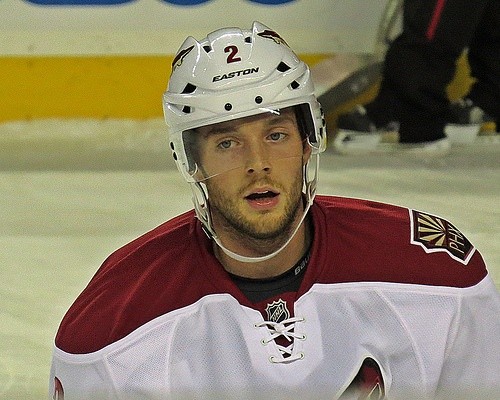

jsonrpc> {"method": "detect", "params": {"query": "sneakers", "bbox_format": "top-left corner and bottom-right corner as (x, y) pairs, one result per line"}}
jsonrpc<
(334, 73), (449, 155)
(443, 81), (499, 154)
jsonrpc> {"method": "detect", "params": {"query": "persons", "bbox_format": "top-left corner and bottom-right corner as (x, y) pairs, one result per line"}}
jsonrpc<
(49, 21), (500, 399)
(332, 0), (499, 156)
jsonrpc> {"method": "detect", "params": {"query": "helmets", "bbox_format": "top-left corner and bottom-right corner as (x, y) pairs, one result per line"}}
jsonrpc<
(163, 20), (328, 196)
